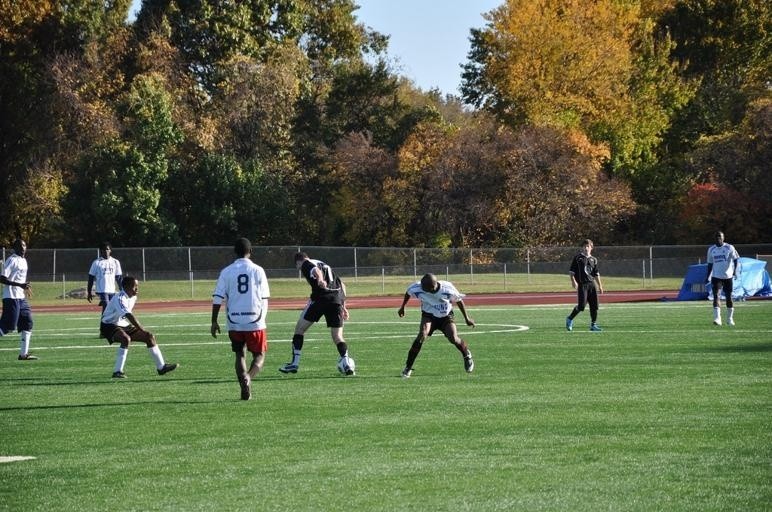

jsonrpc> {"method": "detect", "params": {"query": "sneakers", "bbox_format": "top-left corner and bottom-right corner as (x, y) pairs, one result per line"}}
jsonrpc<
(713, 317), (722, 326)
(111, 371), (129, 379)
(344, 365), (353, 375)
(279, 362), (299, 373)
(725, 317), (736, 326)
(18, 354), (38, 360)
(247, 362), (261, 379)
(463, 349), (474, 372)
(589, 323), (602, 331)
(156, 362), (180, 375)
(403, 366), (413, 378)
(564, 316), (573, 331)
(239, 373), (251, 400)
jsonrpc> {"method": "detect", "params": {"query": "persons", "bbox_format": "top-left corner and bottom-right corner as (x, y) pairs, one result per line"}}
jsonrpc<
(705, 230), (740, 326)
(278, 251), (356, 376)
(397, 272), (477, 378)
(566, 238), (605, 331)
(86, 241), (124, 310)
(99, 276), (180, 379)
(210, 236), (271, 401)
(0, 239), (38, 360)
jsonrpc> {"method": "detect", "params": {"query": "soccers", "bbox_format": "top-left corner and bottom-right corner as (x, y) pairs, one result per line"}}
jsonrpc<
(338, 355), (356, 375)
